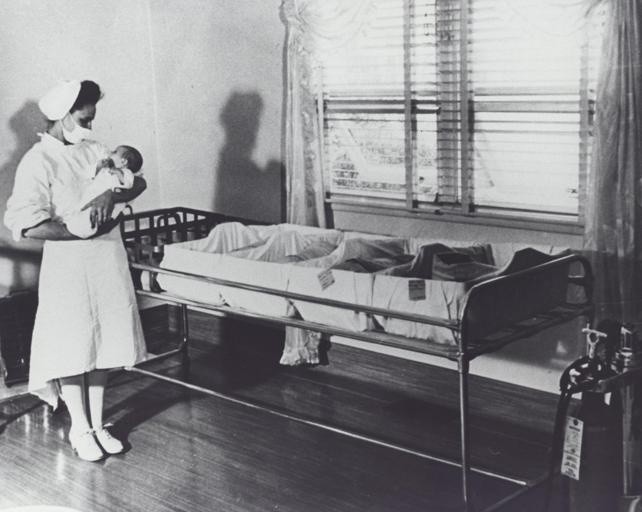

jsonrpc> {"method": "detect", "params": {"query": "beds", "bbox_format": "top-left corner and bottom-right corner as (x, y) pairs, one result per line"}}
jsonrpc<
(121, 206), (593, 512)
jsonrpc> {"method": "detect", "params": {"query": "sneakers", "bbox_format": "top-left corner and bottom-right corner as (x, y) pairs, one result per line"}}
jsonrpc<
(68, 427), (104, 462)
(94, 427), (124, 455)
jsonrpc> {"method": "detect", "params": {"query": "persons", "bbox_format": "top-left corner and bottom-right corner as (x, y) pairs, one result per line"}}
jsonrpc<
(66, 145), (143, 239)
(4, 79), (148, 462)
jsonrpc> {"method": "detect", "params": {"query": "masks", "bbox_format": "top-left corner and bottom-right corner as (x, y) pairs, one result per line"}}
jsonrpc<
(60, 112), (93, 145)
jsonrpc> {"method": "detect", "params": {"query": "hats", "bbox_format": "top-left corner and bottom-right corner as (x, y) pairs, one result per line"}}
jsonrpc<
(38, 81), (82, 123)
